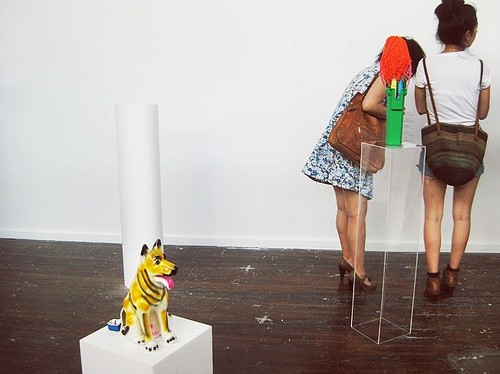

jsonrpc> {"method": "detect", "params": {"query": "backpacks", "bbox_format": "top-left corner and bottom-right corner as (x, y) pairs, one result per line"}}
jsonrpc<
(421, 120), (488, 187)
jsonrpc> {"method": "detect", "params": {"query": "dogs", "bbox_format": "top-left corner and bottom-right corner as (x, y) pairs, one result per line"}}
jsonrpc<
(120, 239), (179, 351)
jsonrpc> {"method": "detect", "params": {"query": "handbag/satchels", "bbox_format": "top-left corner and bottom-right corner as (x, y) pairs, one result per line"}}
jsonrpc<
(327, 71), (387, 174)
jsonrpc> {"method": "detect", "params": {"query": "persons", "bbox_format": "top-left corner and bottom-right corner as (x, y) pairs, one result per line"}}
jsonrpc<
(414, 0), (491, 302)
(302, 36), (426, 291)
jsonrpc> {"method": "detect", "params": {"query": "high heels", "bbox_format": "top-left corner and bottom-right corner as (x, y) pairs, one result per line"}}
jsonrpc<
(427, 277), (441, 301)
(442, 270), (458, 296)
(349, 269), (376, 292)
(338, 258), (354, 281)
